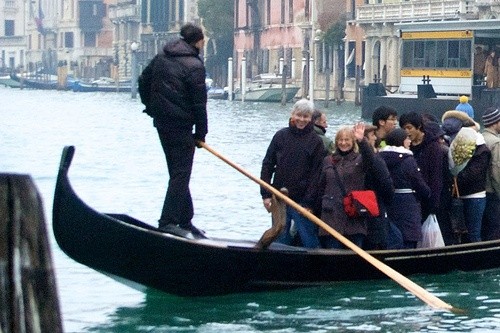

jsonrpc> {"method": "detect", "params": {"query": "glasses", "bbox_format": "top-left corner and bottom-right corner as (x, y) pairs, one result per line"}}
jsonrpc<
(439, 135), (444, 141)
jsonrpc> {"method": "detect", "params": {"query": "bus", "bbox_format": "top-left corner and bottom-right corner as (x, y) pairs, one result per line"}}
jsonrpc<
(395, 20), (500, 95)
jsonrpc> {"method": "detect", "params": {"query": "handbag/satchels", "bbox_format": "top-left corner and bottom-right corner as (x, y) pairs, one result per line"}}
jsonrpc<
(342, 190), (379, 218)
(416, 214), (444, 248)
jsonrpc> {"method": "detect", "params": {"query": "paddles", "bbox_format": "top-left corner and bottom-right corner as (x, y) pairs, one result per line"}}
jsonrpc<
(142, 108), (468, 313)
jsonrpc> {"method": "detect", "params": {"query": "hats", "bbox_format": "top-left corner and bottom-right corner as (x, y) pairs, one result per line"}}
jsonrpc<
(425, 121), (445, 138)
(482, 107), (500, 126)
(354, 125), (378, 130)
(457, 95), (474, 118)
(181, 23), (204, 43)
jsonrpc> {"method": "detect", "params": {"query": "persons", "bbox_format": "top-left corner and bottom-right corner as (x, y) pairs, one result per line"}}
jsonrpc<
(138, 24), (209, 236)
(474, 46), (500, 89)
(260, 95), (500, 248)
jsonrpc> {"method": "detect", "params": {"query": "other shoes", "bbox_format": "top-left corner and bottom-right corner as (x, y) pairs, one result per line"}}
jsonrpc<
(180, 222), (202, 237)
(159, 224), (187, 236)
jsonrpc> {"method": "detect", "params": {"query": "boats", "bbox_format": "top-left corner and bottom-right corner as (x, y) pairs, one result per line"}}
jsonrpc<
(235, 72), (301, 101)
(51, 144), (495, 300)
(0, 67), (57, 88)
